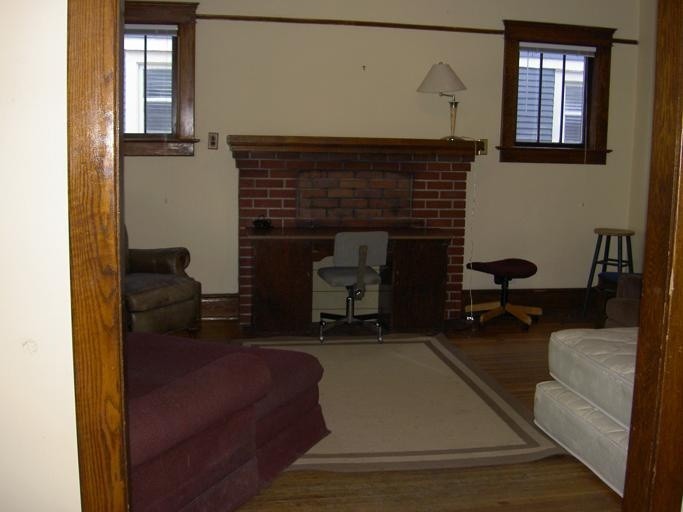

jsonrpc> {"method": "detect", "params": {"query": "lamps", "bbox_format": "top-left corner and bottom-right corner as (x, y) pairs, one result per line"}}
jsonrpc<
(414, 61), (467, 141)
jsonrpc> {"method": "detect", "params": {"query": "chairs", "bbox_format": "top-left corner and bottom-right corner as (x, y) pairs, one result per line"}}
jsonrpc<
(604, 271), (641, 327)
(317, 231), (388, 344)
(123, 223), (202, 339)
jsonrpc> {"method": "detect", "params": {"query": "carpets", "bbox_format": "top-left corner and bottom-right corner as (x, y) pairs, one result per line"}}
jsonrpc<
(228, 327), (569, 474)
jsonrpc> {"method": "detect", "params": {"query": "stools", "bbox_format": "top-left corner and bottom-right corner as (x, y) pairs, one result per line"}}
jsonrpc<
(464, 258), (544, 331)
(579, 227), (635, 320)
